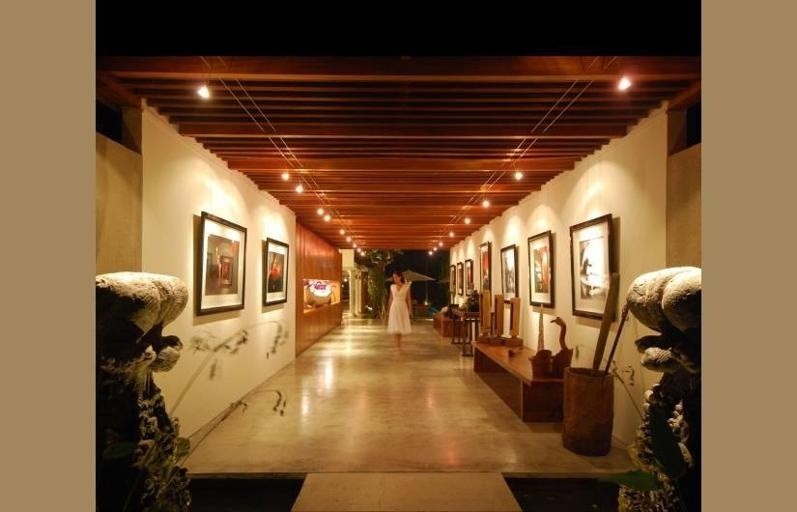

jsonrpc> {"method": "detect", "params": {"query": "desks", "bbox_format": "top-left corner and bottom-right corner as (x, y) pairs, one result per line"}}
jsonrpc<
(452, 308), (480, 349)
(470, 340), (563, 421)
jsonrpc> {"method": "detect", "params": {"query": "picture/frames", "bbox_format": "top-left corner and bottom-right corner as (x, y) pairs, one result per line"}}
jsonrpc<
(568, 213), (615, 322)
(200, 211), (248, 316)
(528, 230), (553, 308)
(478, 241), (492, 295)
(449, 259), (474, 297)
(498, 245), (518, 304)
(264, 237), (291, 307)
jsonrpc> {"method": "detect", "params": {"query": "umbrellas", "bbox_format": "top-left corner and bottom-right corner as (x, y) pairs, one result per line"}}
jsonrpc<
(384, 269), (438, 284)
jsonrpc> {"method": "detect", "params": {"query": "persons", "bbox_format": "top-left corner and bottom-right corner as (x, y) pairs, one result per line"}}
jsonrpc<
(380, 269), (415, 354)
(269, 254), (282, 291)
